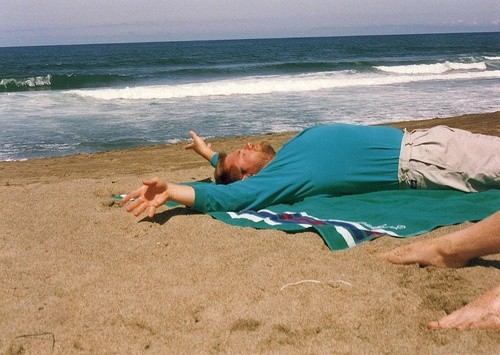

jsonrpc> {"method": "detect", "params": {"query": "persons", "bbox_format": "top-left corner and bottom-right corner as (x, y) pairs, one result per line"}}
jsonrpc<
(385, 205), (500, 330)
(122, 119), (500, 221)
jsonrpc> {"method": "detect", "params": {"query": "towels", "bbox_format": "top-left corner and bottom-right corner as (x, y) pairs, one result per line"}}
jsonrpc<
(111, 178), (500, 251)
